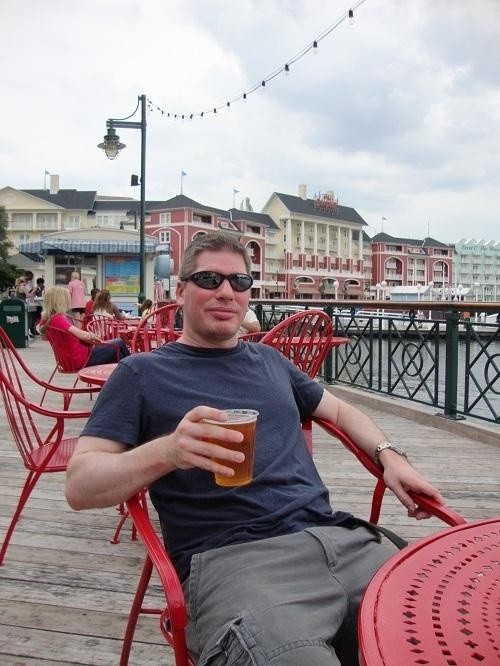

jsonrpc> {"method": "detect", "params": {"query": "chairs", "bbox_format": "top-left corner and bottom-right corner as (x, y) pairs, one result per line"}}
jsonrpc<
(114, 412), (468, 666)
(0, 327), (151, 566)
(36, 303), (286, 412)
(254, 310), (336, 460)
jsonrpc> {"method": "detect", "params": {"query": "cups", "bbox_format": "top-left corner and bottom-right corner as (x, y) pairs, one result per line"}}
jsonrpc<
(209, 407), (260, 488)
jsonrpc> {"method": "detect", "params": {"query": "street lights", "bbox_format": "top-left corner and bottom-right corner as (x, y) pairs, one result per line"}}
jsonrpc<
(333, 278), (435, 323)
(96, 94), (147, 319)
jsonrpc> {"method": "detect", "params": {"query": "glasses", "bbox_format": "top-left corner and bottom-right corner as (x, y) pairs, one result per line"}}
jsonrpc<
(181, 271), (254, 293)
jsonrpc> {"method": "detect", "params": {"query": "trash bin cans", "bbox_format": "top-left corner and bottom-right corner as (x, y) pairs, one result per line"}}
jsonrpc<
(0, 298), (27, 348)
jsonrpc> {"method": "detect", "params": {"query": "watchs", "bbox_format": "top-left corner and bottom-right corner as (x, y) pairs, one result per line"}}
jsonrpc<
(374, 440), (411, 471)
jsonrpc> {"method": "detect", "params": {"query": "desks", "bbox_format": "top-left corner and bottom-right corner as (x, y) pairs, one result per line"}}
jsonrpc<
(356, 517), (500, 663)
(266, 334), (350, 376)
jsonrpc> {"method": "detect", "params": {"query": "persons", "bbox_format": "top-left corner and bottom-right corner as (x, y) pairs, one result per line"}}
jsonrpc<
(168, 299), (184, 330)
(23, 269), (41, 336)
(81, 287), (102, 332)
(61, 230), (447, 666)
(13, 275), (38, 339)
(234, 304), (263, 343)
(66, 271), (90, 326)
(141, 299), (156, 322)
(37, 282), (133, 374)
(34, 277), (46, 324)
(89, 286), (128, 341)
